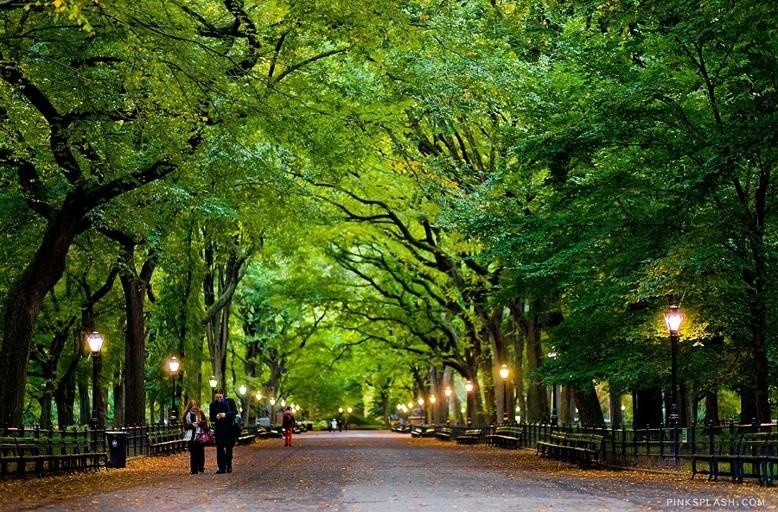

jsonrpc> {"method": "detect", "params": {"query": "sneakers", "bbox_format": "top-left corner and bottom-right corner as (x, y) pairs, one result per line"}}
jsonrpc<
(189, 468), (204, 474)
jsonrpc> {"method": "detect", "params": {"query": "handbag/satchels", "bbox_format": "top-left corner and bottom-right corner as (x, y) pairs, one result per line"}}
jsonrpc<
(196, 431), (217, 447)
(231, 416), (243, 437)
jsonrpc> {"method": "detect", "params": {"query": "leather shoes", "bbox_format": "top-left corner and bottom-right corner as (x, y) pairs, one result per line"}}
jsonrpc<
(216, 469), (225, 474)
(227, 464), (232, 472)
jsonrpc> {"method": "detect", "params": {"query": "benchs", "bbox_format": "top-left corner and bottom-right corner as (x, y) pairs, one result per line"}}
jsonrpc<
(410, 426), (605, 467)
(146, 429), (189, 455)
(1, 425), (109, 478)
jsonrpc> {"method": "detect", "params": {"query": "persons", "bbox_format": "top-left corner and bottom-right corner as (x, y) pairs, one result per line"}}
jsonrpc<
(330, 418), (337, 432)
(281, 406), (295, 448)
(182, 399), (209, 475)
(328, 420), (332, 432)
(206, 388), (238, 476)
(336, 419), (342, 432)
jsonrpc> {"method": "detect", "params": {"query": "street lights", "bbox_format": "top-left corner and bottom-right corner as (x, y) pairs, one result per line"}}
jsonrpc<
(347, 407), (353, 430)
(548, 346), (560, 425)
(664, 303), (686, 452)
(238, 385), (300, 428)
(500, 363), (509, 424)
(338, 407), (344, 416)
(208, 375), (217, 401)
(169, 355), (181, 425)
(396, 394), (437, 426)
(86, 329), (103, 429)
(444, 386), (453, 426)
(464, 381), (474, 427)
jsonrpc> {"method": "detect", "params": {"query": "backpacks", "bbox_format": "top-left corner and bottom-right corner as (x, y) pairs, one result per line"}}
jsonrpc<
(286, 414), (292, 427)
